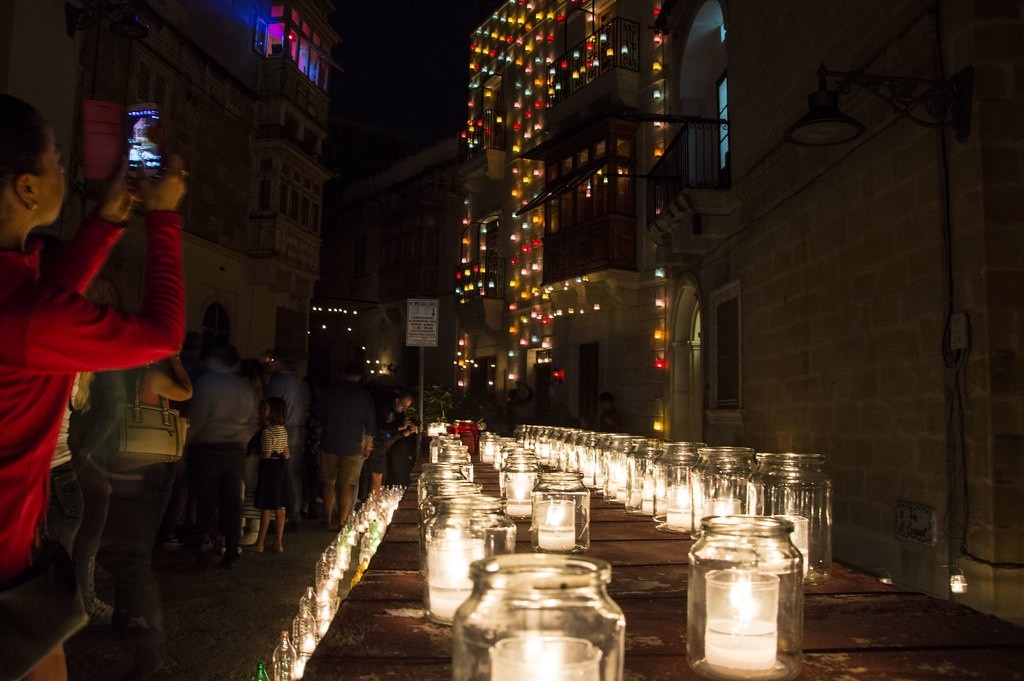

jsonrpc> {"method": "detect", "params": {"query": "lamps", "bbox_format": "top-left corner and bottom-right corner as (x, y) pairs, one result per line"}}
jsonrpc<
(65, 0), (149, 40)
(783, 61), (976, 148)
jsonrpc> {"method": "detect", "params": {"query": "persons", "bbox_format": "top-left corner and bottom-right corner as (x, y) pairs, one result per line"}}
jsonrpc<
(170, 330), (311, 570)
(0, 93), (186, 681)
(369, 392), (418, 492)
(599, 392), (625, 433)
(315, 359), (377, 530)
(49, 357), (194, 681)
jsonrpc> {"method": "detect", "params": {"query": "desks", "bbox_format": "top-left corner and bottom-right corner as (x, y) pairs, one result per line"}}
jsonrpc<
(298, 455), (1024, 681)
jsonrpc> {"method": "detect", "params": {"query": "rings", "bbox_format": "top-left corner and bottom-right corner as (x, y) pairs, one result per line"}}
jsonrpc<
(179, 168), (186, 175)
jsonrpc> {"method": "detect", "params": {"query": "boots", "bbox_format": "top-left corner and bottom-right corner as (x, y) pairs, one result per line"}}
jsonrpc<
(72, 553), (114, 624)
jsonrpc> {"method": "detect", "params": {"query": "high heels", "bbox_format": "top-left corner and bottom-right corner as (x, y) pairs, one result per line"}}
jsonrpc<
(109, 609), (167, 640)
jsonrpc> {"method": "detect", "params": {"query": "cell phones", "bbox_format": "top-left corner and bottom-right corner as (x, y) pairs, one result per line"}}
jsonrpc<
(125, 102), (165, 178)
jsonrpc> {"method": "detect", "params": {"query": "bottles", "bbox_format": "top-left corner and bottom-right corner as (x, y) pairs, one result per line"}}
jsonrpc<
(416, 423), (833, 681)
(251, 483), (404, 681)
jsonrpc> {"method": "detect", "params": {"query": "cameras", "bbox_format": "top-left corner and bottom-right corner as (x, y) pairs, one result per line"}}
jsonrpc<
(407, 417), (421, 427)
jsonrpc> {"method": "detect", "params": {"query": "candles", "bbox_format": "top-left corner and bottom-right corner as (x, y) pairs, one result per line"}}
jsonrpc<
(428, 445), (808, 681)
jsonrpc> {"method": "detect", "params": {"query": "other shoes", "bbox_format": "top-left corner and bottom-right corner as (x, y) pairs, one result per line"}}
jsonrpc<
(163, 495), (367, 570)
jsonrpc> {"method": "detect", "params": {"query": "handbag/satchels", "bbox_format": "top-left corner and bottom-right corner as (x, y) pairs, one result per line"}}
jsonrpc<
(115, 373), (184, 463)
